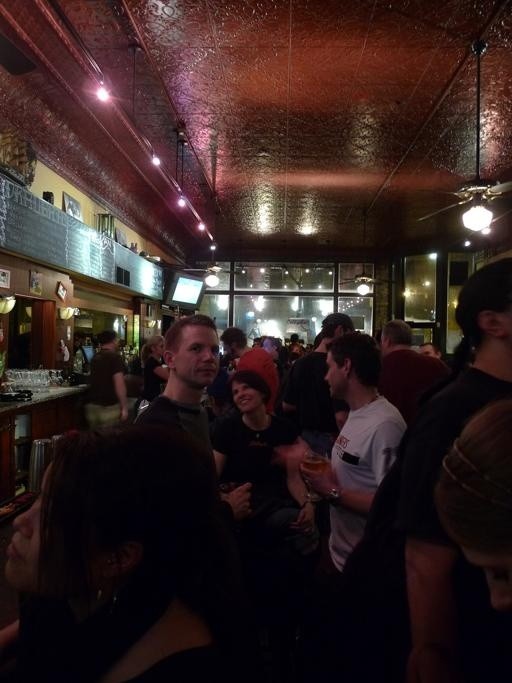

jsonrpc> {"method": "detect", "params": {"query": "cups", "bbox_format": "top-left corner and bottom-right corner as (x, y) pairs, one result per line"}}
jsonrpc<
(219, 481), (242, 492)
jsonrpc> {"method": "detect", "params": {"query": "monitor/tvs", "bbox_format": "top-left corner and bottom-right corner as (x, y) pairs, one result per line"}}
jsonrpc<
(164, 271), (207, 311)
(80, 345), (95, 364)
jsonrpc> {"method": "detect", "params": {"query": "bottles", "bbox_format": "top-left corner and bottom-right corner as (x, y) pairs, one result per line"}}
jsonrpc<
(28, 435), (62, 493)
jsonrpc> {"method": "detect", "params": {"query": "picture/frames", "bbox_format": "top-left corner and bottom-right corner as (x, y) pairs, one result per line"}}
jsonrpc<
(0, 268), (10, 289)
(62, 192), (84, 223)
(56, 281), (68, 301)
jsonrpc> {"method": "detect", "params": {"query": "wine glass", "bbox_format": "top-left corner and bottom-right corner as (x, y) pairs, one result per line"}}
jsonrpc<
(0, 369), (63, 402)
(302, 448), (328, 502)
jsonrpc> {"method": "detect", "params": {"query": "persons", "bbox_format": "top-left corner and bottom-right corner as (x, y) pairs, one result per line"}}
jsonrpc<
(2, 258), (512, 682)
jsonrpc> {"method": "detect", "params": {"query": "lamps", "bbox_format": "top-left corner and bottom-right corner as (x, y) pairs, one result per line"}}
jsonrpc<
(354, 262), (374, 295)
(461, 40), (494, 232)
(286, 318), (309, 344)
(204, 252), (221, 287)
